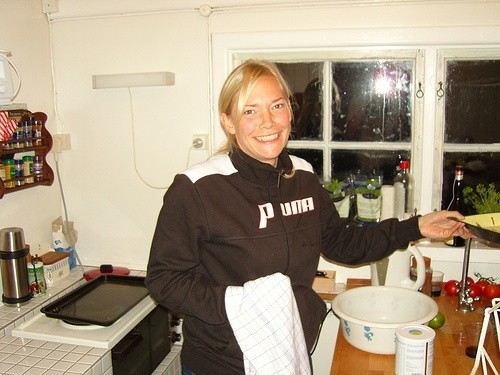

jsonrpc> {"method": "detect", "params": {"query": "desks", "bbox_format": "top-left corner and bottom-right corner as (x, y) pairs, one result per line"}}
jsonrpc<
(329, 278), (500, 375)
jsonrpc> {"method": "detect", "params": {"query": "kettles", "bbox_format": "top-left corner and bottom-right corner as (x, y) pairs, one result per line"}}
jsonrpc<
(370, 243), (426, 291)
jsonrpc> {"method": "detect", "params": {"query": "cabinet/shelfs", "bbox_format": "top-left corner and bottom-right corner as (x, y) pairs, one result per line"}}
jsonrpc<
(0, 109), (54, 200)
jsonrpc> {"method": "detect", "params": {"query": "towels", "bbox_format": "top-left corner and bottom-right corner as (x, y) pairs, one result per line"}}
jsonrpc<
(224, 272), (312, 375)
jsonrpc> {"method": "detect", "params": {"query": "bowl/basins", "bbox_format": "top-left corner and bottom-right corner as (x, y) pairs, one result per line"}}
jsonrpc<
(332, 286), (439, 354)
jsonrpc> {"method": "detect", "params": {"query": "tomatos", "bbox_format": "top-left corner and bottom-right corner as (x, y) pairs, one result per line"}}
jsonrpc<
(427, 311), (445, 329)
(444, 276), (500, 298)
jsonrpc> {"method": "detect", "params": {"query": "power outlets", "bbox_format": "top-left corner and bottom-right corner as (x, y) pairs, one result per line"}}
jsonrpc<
(192, 134), (207, 150)
(52, 134), (71, 150)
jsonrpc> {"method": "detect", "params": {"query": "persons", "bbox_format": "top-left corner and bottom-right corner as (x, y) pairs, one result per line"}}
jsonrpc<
(288, 75), (392, 181)
(145, 60), (477, 375)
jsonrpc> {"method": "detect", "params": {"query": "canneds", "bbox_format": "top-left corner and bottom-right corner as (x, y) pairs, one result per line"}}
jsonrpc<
(0, 121), (45, 189)
(27, 261), (47, 297)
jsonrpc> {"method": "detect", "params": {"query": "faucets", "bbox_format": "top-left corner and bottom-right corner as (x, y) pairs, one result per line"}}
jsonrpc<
(454, 237), (481, 314)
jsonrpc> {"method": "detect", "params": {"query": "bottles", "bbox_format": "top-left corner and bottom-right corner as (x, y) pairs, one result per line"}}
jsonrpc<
(444, 166), (466, 247)
(410, 256), (432, 298)
(4, 122), (24, 150)
(24, 121), (32, 147)
(34, 156), (43, 181)
(33, 121), (42, 146)
(22, 156), (35, 183)
(394, 161), (413, 220)
(15, 160), (24, 186)
(4, 160), (15, 188)
(0, 161), (6, 188)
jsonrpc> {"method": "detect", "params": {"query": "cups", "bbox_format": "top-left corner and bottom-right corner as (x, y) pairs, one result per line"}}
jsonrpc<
(463, 324), (488, 358)
(432, 271), (444, 297)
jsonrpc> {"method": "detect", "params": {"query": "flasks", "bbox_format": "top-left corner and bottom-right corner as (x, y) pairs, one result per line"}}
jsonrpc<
(0, 227), (33, 307)
